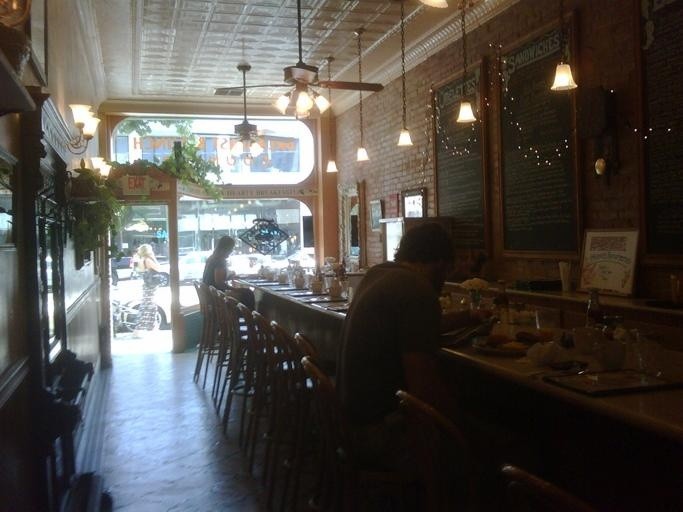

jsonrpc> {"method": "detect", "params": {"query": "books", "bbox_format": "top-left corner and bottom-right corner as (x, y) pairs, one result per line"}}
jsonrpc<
(440, 314), (499, 348)
(541, 367), (683, 398)
(234, 272), (351, 311)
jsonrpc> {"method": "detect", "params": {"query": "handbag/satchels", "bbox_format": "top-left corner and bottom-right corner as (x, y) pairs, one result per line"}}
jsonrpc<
(143, 269), (163, 289)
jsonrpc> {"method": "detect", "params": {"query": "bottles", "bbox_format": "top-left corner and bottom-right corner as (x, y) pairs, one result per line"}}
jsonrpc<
(493, 280), (510, 324)
(586, 289), (603, 332)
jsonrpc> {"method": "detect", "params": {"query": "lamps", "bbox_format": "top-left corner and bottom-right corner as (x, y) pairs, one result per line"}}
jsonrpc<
(65, 103), (112, 181)
(0, 0), (33, 80)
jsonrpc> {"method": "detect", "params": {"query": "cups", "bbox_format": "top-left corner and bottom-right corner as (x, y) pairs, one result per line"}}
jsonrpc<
(558, 260), (573, 291)
(571, 327), (597, 354)
(261, 270), (341, 298)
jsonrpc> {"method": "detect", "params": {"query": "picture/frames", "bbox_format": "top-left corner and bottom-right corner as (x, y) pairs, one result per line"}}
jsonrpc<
(575, 228), (640, 297)
(369, 200), (383, 232)
(400, 187), (426, 218)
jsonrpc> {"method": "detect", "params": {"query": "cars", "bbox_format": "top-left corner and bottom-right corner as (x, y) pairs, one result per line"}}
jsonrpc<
(111, 257), (138, 280)
(115, 285), (188, 330)
(154, 248), (271, 287)
(271, 248), (314, 268)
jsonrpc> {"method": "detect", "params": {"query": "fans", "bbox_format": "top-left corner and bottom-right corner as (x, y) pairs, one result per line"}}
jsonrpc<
(213, 1), (385, 92)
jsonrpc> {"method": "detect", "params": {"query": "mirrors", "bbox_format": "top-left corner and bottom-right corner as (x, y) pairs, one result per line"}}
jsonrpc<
(337, 177), (368, 270)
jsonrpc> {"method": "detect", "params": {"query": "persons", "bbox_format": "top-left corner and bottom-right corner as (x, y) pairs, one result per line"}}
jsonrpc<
(199, 235), (255, 317)
(127, 249), (141, 279)
(135, 244), (162, 331)
(110, 234), (122, 291)
(335, 221), (492, 511)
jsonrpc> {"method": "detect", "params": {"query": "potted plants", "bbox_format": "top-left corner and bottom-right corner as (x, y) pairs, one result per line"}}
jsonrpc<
(43, 172), (134, 262)
(257, 259), (346, 297)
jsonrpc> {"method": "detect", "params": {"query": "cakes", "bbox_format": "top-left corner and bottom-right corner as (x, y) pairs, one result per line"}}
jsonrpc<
(526, 341), (567, 367)
